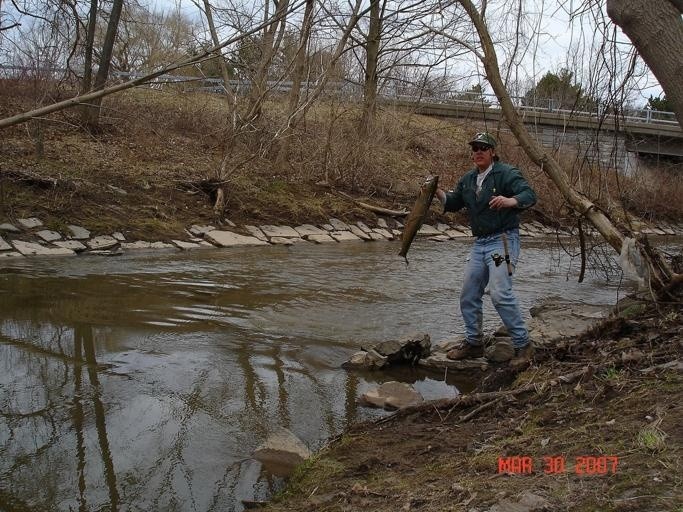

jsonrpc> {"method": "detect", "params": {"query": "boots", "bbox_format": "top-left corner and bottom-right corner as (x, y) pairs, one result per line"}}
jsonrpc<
(446, 341), (484, 360)
(508, 342), (534, 369)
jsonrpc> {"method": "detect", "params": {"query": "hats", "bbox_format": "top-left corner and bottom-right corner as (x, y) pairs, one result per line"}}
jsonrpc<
(468, 131), (497, 147)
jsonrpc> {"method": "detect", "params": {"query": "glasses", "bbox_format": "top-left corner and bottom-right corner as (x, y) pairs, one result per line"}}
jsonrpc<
(472, 144), (492, 152)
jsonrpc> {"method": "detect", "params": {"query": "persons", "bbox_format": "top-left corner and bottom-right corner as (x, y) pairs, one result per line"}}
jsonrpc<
(435, 131), (537, 369)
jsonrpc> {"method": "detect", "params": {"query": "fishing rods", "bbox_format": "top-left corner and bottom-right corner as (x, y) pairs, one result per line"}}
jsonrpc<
(469, 0), (514, 278)
